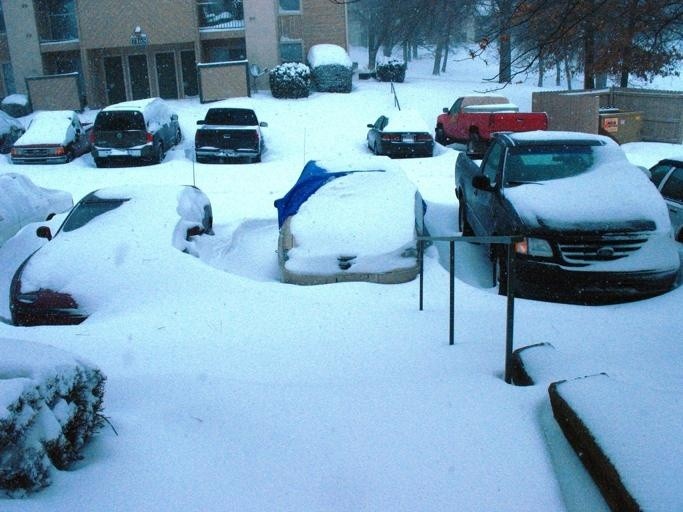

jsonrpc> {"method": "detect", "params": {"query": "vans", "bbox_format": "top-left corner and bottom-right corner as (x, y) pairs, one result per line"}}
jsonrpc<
(91, 96), (182, 165)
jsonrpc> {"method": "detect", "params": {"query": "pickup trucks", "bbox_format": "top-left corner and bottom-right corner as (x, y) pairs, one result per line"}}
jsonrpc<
(455, 131), (676, 300)
(434, 96), (547, 152)
(9, 113), (92, 167)
(193, 107), (266, 166)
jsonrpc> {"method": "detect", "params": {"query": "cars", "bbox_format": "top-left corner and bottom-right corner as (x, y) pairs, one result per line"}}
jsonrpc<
(8, 182), (214, 327)
(275, 155), (425, 287)
(363, 115), (434, 158)
(0, 173), (72, 237)
(644, 155), (682, 241)
